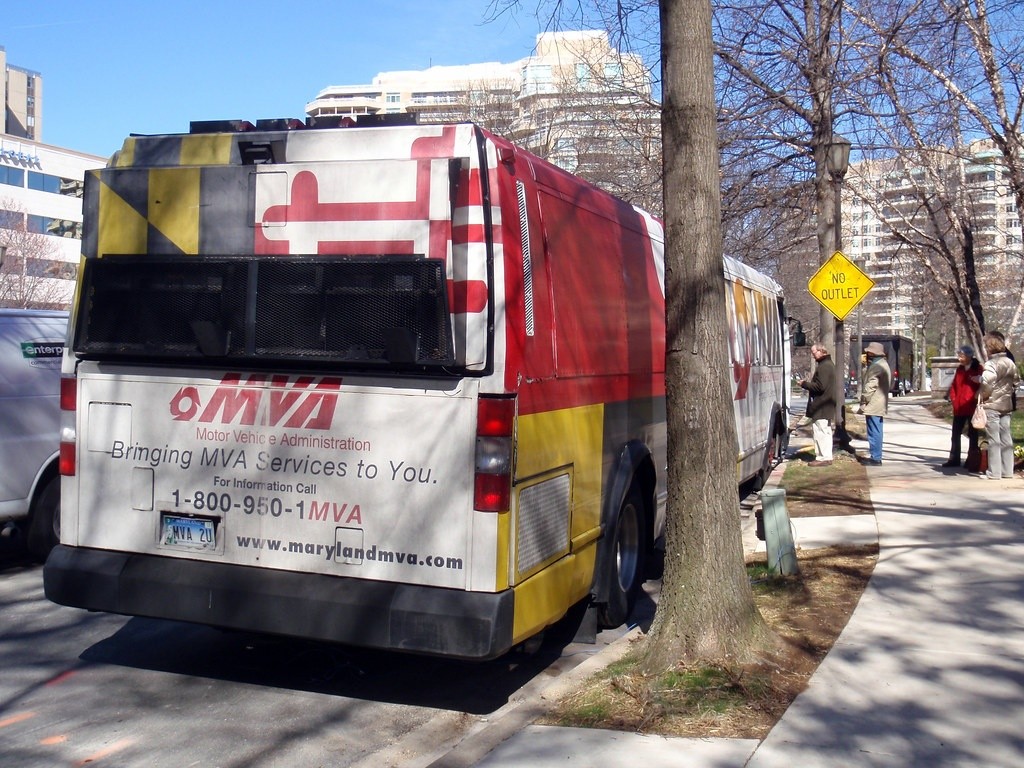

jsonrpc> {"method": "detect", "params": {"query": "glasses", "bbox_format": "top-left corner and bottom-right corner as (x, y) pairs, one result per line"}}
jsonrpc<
(866, 352), (871, 357)
(811, 351), (818, 356)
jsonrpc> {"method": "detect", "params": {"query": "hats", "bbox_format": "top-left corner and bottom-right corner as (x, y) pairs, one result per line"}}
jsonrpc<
(864, 342), (885, 355)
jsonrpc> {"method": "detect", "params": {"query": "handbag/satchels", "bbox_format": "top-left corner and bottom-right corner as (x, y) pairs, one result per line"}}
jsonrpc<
(971, 391), (987, 429)
(969, 441), (989, 474)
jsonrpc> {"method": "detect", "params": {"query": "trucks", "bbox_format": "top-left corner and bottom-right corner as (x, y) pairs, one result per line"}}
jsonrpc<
(848, 334), (916, 395)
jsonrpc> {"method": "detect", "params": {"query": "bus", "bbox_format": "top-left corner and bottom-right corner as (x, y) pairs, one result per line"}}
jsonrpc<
(41, 113), (806, 661)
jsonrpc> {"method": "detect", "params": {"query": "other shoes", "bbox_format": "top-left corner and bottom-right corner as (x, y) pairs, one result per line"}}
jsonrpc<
(963, 462), (968, 468)
(861, 459), (882, 466)
(942, 459), (961, 467)
(808, 460), (832, 466)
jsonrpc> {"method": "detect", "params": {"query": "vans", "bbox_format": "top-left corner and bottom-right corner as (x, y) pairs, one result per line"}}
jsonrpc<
(1, 308), (76, 560)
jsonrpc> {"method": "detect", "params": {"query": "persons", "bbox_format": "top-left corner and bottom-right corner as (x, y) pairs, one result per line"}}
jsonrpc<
(942, 330), (1017, 479)
(858, 341), (892, 465)
(798, 344), (838, 465)
